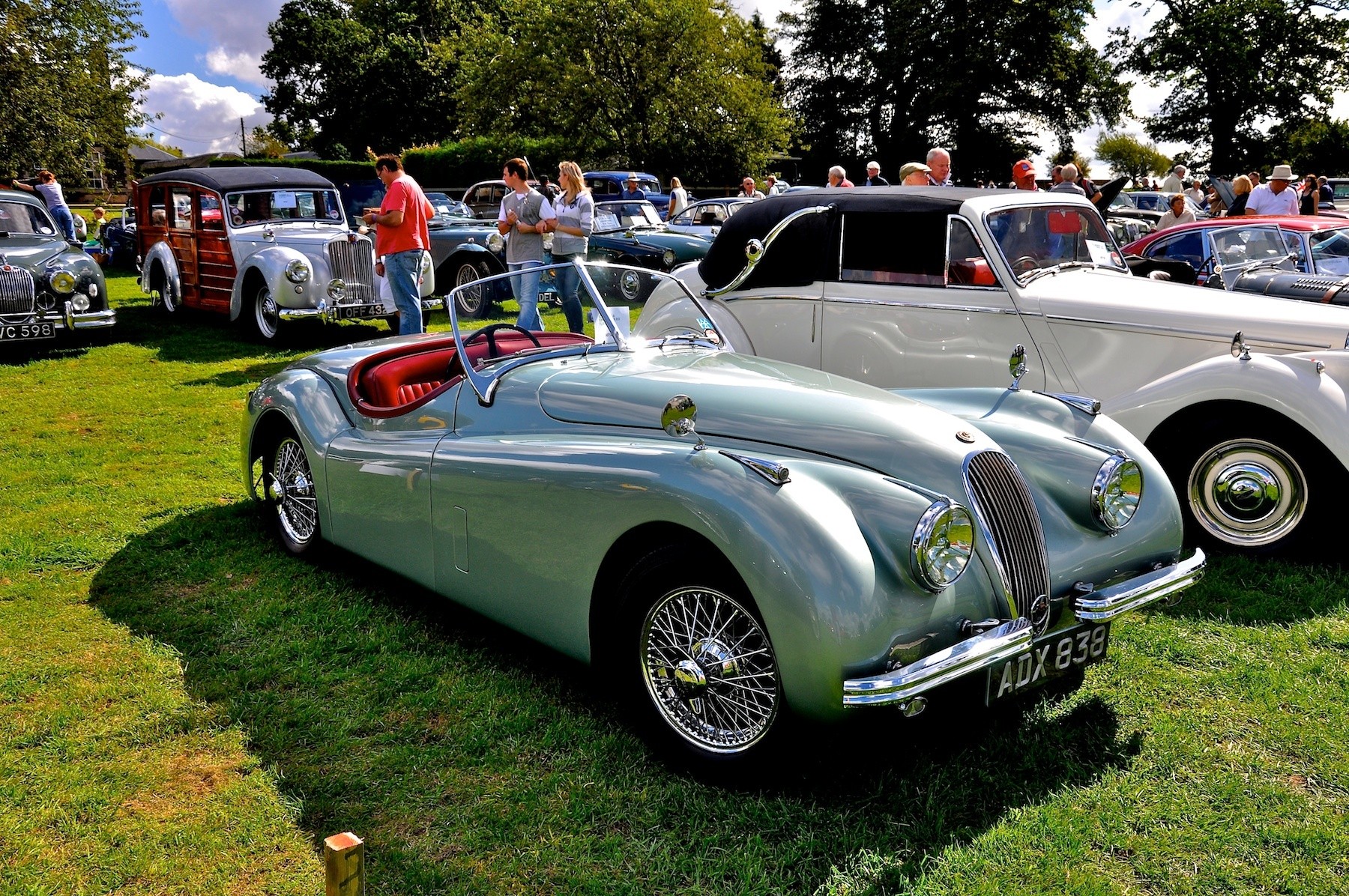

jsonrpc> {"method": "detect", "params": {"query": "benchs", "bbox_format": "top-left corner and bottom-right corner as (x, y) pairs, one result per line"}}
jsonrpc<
(358, 336), (591, 410)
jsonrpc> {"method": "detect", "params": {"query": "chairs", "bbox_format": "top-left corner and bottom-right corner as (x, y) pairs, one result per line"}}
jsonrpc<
(701, 212), (716, 226)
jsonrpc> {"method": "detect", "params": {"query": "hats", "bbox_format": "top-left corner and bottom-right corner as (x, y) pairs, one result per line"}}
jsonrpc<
(1013, 160), (1037, 179)
(900, 162), (932, 182)
(1009, 182), (1016, 188)
(625, 171), (641, 181)
(1266, 165), (1299, 180)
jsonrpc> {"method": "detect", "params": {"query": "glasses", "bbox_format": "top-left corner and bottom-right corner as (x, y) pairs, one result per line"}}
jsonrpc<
(1306, 180), (1311, 182)
(1280, 179), (1292, 184)
(378, 171), (382, 179)
(746, 183), (754, 185)
(867, 168), (876, 171)
(1250, 177), (1255, 180)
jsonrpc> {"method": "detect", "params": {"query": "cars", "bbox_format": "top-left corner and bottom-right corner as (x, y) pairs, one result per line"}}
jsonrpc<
(93, 171), (768, 323)
(988, 177), (1349, 311)
(0, 189), (118, 347)
(133, 167), (445, 346)
(632, 185), (1349, 567)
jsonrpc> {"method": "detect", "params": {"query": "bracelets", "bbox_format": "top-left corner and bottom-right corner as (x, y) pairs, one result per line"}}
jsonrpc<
(375, 258), (382, 266)
(372, 214), (377, 223)
(555, 221), (560, 229)
(505, 220), (511, 228)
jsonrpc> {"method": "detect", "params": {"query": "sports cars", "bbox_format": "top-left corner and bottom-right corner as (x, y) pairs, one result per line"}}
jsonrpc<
(240, 256), (1210, 792)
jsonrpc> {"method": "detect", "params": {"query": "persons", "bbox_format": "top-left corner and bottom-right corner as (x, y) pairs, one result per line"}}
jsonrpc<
(926, 148), (956, 187)
(536, 161), (595, 335)
(12, 170), (76, 252)
(621, 172), (646, 216)
(826, 165), (855, 187)
(996, 159), (1103, 262)
(899, 162), (933, 186)
(92, 206), (108, 242)
(498, 158), (556, 331)
(737, 174), (780, 200)
(666, 176), (691, 221)
(863, 161), (889, 186)
(151, 209), (166, 228)
(988, 180), (997, 188)
(977, 180), (985, 188)
(536, 174), (555, 207)
(362, 154), (435, 337)
(1155, 164), (1334, 233)
(1132, 177), (1158, 190)
